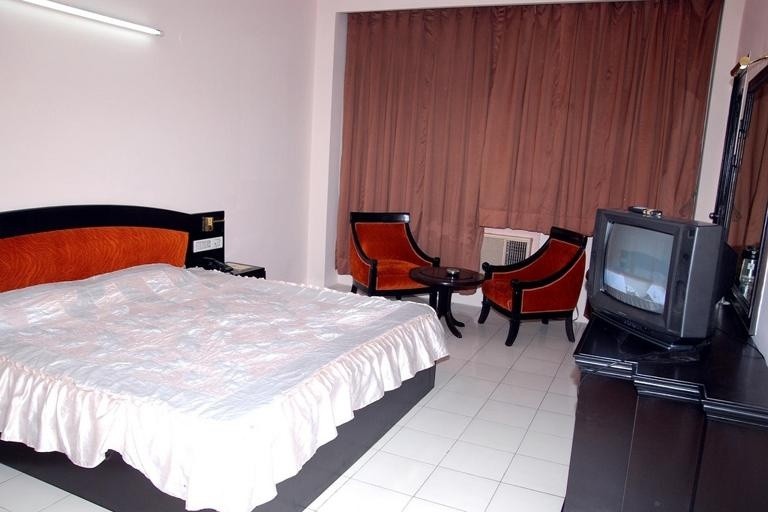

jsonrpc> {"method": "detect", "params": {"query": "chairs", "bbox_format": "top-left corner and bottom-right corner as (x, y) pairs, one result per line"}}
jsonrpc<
(478, 226), (589, 348)
(347, 211), (442, 312)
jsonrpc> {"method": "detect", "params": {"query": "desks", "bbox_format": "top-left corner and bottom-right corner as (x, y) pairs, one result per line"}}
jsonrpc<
(407, 266), (486, 339)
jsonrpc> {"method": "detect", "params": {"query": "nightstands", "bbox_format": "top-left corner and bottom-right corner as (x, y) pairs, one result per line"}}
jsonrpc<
(216, 262), (266, 277)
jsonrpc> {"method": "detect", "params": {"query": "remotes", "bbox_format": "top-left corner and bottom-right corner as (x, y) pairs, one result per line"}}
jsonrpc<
(630, 206), (662, 216)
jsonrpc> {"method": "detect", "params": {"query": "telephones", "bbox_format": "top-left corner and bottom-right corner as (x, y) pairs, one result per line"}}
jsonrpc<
(202, 257), (234, 275)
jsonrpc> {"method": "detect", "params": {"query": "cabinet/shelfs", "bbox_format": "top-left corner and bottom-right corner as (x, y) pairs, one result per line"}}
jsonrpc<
(559, 295), (767, 511)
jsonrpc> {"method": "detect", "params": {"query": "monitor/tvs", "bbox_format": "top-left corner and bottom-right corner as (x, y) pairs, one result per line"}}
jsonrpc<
(587, 207), (726, 351)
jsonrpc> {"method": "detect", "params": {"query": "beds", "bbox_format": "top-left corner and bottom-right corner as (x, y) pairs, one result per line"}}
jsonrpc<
(0, 205), (450, 512)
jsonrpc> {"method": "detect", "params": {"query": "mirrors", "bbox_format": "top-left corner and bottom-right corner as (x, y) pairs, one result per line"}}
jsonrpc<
(718, 57), (768, 338)
